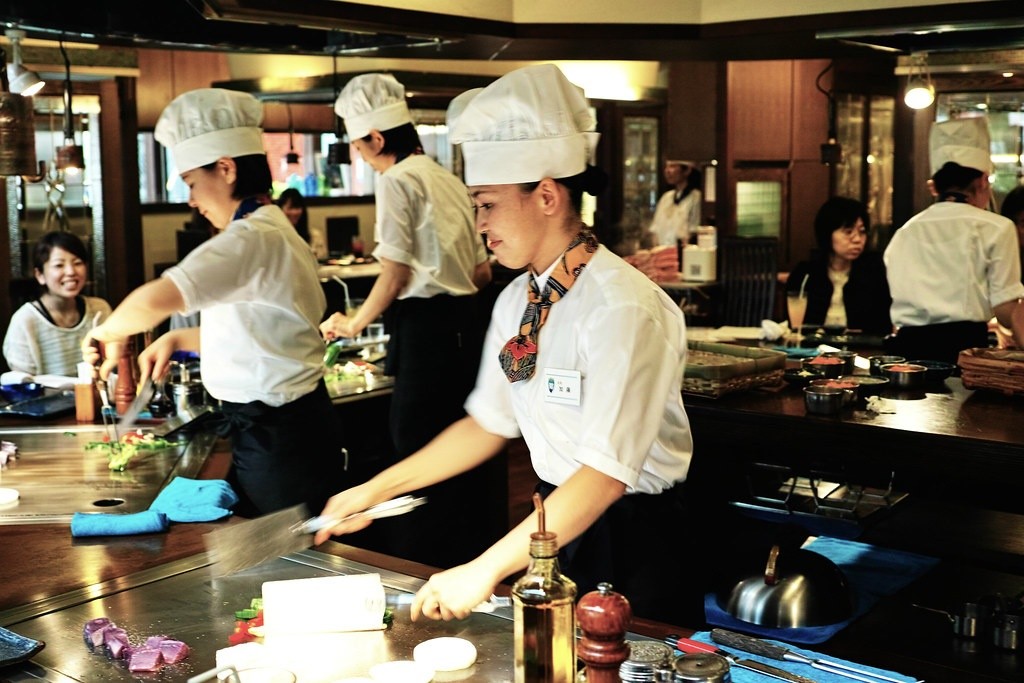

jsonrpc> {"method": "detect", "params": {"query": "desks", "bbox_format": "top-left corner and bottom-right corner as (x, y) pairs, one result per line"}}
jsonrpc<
(315, 260), (379, 279)
(661, 278), (719, 304)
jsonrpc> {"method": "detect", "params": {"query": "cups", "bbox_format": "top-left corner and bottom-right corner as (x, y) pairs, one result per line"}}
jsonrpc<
(344, 300), (366, 345)
(786, 292), (808, 340)
(364, 312), (384, 354)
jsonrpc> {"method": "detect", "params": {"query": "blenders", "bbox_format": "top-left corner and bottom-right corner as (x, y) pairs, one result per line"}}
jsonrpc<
(680, 226), (717, 283)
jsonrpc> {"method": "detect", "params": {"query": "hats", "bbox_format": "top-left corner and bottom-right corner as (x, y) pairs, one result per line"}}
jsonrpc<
(927, 117), (993, 179)
(445, 62), (601, 186)
(154, 88), (266, 177)
(333, 74), (413, 142)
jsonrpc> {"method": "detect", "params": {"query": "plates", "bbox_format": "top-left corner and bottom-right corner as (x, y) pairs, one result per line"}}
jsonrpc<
(0, 626), (45, 665)
(782, 368), (824, 386)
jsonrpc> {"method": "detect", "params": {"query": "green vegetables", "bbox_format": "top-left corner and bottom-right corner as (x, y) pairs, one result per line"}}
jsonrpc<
(64, 431), (188, 472)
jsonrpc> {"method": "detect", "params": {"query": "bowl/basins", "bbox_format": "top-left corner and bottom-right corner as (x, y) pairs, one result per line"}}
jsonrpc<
(797, 354), (956, 412)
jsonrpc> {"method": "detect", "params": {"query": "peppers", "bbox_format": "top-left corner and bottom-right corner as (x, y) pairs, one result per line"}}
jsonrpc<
(228, 609), (263, 642)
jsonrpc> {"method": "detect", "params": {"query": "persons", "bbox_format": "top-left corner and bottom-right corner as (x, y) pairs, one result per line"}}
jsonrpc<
(314, 62), (707, 629)
(645, 158), (702, 246)
(318, 71), (493, 567)
(79, 86), (340, 519)
(277, 187), (327, 260)
(3, 230), (113, 378)
(880, 117), (1024, 359)
(783, 196), (894, 338)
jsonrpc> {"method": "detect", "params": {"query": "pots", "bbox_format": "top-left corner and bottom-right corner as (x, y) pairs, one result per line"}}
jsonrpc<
(908, 597), (1024, 651)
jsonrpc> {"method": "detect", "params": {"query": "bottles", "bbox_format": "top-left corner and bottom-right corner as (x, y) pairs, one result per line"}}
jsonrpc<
(509, 533), (578, 683)
(616, 634), (738, 683)
(173, 382), (205, 417)
(148, 370), (173, 418)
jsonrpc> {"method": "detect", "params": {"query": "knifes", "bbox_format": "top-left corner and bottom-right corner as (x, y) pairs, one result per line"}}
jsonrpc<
(665, 636), (816, 683)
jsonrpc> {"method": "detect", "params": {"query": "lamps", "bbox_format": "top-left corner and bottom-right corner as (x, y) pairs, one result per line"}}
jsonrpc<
(56, 42), (86, 170)
(904, 51), (935, 110)
(816, 62), (843, 163)
(285, 102), (300, 165)
(328, 55), (352, 166)
(4, 30), (45, 98)
(0, 93), (36, 177)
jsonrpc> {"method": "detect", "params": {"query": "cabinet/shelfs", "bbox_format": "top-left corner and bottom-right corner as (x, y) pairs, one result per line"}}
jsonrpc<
(718, 58), (834, 275)
